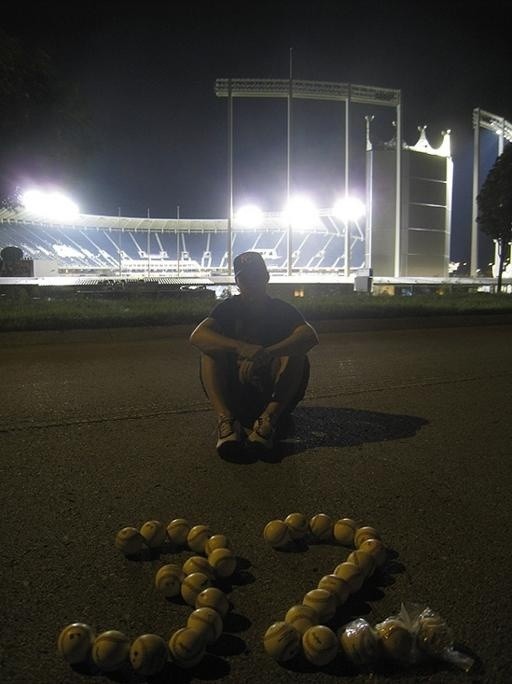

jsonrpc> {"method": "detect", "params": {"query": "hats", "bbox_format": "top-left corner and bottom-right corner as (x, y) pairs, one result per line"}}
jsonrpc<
(233, 252), (265, 278)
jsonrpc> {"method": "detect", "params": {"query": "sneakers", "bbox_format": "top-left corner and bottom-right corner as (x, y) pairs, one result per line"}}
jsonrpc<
(214, 414), (242, 451)
(245, 415), (280, 452)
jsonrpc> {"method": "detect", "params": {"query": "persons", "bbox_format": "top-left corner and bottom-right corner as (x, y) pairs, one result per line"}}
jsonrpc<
(187, 251), (319, 453)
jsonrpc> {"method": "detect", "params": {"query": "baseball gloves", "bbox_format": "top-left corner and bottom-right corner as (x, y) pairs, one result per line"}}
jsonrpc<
(238, 350), (280, 389)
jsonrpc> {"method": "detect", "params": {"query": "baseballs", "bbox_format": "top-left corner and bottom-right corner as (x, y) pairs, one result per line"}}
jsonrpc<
(262, 512), (445, 660)
(60, 513), (232, 668)
